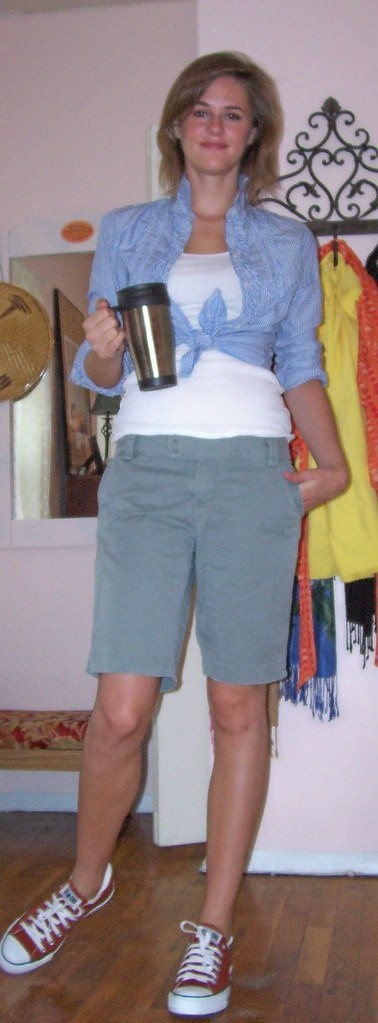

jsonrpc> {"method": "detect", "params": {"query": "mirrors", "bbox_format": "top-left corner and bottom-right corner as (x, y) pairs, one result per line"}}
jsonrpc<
(9, 250), (120, 522)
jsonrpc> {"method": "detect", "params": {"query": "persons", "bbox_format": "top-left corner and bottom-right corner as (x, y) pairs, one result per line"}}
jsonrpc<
(0, 51), (351, 1016)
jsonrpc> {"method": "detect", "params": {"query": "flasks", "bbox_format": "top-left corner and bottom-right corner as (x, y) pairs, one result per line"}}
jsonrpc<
(110, 281), (178, 391)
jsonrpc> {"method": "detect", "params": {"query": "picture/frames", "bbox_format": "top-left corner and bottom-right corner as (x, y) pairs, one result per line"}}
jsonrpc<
(52, 287), (95, 475)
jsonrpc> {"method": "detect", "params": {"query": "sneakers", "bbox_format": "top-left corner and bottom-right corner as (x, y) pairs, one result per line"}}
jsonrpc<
(166, 920), (234, 1015)
(0, 862), (115, 975)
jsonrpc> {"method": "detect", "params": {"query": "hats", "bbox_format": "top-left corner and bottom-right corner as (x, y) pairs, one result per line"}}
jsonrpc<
(0, 281), (51, 402)
